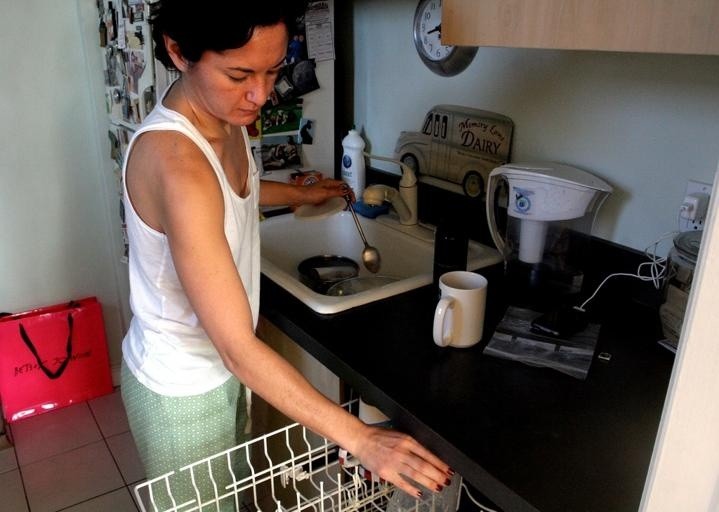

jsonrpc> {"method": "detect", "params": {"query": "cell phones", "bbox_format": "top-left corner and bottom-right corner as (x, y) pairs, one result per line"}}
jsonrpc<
(531, 305), (590, 339)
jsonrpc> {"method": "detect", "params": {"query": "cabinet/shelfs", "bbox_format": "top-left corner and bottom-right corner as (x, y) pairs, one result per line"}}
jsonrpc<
(134, 316), (502, 511)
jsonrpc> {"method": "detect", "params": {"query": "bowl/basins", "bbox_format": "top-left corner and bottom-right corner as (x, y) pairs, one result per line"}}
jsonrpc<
(294, 252), (400, 295)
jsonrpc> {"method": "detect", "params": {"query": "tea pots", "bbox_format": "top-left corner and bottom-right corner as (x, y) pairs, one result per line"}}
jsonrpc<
(483, 162), (612, 290)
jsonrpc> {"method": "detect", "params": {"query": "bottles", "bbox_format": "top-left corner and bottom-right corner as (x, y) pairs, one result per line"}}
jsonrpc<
(658, 229), (703, 348)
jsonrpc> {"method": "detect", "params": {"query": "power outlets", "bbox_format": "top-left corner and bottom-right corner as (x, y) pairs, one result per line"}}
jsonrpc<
(676, 180), (713, 234)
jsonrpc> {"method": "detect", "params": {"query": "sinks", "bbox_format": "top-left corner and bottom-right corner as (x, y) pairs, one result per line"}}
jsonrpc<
(260, 211), (504, 316)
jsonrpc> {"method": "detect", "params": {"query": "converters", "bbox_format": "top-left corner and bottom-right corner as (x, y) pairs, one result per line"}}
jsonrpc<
(681, 191), (709, 223)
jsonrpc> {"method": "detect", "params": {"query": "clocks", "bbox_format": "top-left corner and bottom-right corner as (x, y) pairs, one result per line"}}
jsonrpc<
(413, 0), (480, 77)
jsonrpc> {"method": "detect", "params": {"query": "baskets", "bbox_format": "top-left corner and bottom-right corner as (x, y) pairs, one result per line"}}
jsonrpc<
(133, 399), (495, 512)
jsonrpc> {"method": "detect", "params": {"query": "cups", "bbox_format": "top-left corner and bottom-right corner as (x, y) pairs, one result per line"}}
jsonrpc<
(433, 271), (488, 351)
(337, 397), (394, 484)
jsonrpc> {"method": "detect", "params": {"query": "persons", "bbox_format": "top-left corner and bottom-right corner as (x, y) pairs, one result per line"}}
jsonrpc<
(120, 1), (455, 512)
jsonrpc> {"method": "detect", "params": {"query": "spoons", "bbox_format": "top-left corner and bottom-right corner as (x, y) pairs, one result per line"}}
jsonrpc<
(345, 191), (382, 275)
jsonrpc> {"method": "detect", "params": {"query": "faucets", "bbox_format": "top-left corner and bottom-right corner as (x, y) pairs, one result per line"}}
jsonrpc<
(360, 151), (420, 227)
